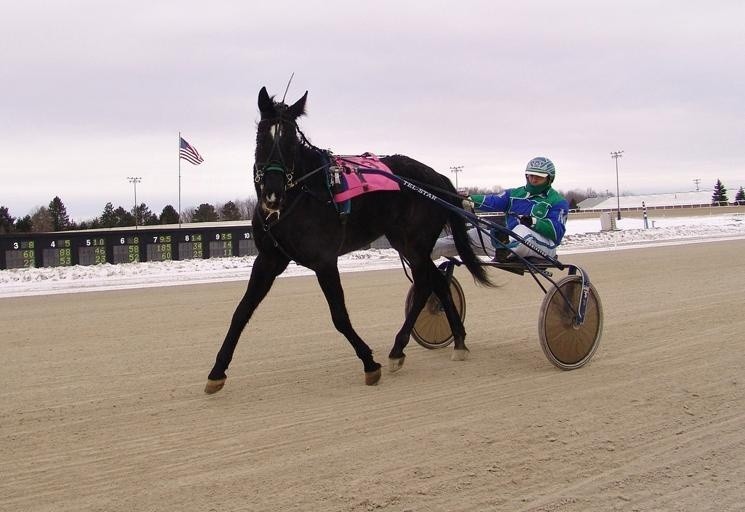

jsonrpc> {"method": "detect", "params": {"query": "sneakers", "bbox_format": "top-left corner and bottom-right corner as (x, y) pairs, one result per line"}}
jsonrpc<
(499, 252), (523, 263)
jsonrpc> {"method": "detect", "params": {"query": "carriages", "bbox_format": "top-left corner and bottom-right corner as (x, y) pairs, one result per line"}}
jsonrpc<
(204, 86), (606, 393)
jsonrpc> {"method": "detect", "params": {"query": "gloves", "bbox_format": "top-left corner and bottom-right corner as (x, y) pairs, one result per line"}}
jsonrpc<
(520, 215), (533, 227)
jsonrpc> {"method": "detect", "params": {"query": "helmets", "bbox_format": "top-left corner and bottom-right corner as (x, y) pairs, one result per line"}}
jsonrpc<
(525, 157), (556, 194)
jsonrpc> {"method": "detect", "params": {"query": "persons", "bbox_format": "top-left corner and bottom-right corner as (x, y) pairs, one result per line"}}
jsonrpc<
(429, 157), (568, 263)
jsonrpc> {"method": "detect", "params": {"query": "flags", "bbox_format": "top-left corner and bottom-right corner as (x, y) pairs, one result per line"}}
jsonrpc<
(179, 138), (203, 167)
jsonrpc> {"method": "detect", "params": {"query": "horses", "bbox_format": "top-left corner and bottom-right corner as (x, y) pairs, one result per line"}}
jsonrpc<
(204, 86), (507, 394)
